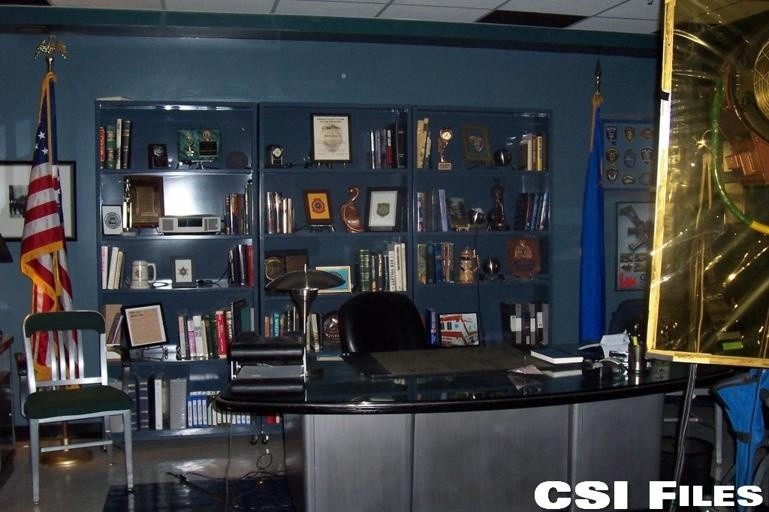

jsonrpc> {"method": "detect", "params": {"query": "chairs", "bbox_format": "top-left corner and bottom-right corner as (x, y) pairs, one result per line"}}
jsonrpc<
(337, 291), (431, 354)
(21, 309), (135, 501)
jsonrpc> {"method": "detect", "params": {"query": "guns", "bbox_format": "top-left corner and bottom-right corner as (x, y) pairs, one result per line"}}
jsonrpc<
(619, 204), (653, 253)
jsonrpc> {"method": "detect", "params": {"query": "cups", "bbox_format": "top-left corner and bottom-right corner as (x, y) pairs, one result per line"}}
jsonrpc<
(628, 343), (649, 373)
(130, 261), (157, 290)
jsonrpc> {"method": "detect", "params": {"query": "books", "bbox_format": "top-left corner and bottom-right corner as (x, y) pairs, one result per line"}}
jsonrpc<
(224, 179), (253, 235)
(517, 132), (544, 172)
(529, 347), (585, 365)
(227, 238), (255, 288)
(514, 191), (549, 231)
(500, 299), (549, 346)
(265, 191), (295, 235)
(100, 244), (126, 290)
(416, 187), (449, 233)
(127, 371), (281, 432)
(98, 116), (132, 171)
(358, 240), (408, 293)
(264, 302), (320, 353)
(178, 298), (255, 359)
(425, 306), (439, 347)
(416, 118), (432, 169)
(416, 241), (456, 285)
(369, 121), (408, 170)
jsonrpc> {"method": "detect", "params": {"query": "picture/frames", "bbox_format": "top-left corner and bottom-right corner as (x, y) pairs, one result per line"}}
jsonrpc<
(0, 160), (78, 243)
(599, 118), (654, 192)
(614, 201), (654, 291)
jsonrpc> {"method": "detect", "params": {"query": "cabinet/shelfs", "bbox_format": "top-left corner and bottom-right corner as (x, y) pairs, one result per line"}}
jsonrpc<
(257, 102), (412, 445)
(95, 100), (260, 450)
(411, 105), (554, 348)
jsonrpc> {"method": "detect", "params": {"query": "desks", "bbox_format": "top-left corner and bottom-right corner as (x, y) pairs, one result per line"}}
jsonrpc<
(213, 346), (748, 512)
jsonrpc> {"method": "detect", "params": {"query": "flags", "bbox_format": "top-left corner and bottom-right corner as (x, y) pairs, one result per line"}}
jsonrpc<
(577, 92), (607, 345)
(18, 70), (84, 393)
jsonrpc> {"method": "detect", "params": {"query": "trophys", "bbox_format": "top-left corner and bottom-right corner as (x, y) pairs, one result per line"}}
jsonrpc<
(437, 128), (455, 171)
